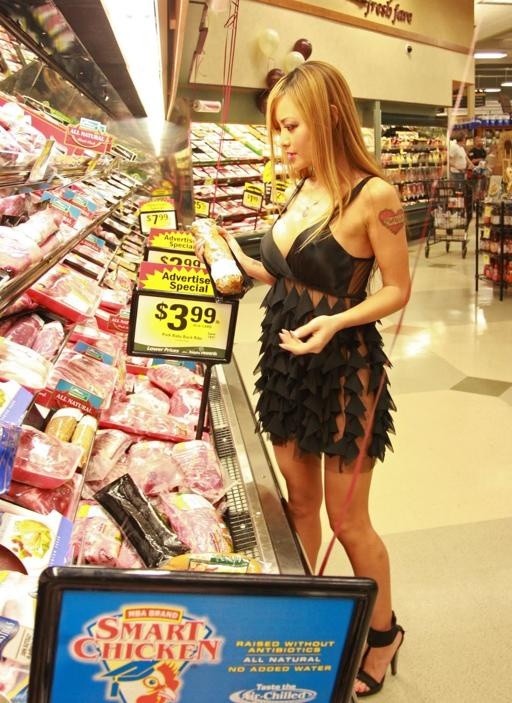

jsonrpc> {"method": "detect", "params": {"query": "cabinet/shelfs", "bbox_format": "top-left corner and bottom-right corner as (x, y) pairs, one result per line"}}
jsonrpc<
(475, 199), (512, 300)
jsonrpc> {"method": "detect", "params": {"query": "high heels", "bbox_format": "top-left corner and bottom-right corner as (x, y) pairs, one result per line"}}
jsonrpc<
(353, 608), (406, 697)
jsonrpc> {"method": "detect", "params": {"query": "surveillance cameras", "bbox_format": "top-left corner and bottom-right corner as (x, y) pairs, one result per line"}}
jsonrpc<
(405, 45), (413, 54)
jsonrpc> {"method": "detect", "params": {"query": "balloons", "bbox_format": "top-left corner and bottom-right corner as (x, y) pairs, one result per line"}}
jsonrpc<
(266, 67), (285, 89)
(259, 27), (278, 59)
(255, 88), (272, 114)
(285, 51), (306, 73)
(295, 38), (312, 60)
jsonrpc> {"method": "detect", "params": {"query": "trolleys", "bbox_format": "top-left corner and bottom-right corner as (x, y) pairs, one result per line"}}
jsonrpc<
(416, 178), (471, 257)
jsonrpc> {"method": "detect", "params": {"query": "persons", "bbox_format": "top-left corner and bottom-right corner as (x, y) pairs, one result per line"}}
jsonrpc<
(467, 134), (486, 166)
(192, 60), (411, 696)
(449, 130), (475, 181)
